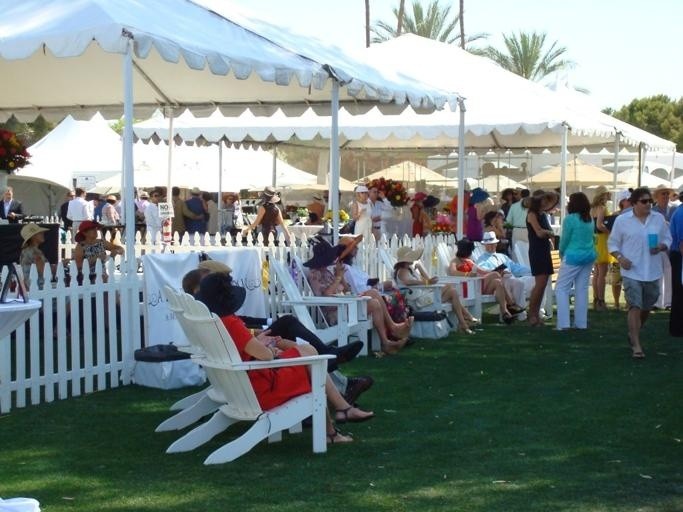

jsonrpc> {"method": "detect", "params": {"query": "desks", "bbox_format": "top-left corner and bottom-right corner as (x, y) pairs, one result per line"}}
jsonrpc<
(287, 225), (325, 240)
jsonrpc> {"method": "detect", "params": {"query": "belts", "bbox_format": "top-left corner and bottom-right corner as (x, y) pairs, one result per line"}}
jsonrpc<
(373, 227), (381, 229)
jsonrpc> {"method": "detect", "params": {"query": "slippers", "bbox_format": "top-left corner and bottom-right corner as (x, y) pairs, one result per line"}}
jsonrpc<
(628, 331), (645, 359)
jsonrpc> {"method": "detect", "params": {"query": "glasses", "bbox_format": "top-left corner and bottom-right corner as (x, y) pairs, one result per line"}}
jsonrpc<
(637, 198), (653, 204)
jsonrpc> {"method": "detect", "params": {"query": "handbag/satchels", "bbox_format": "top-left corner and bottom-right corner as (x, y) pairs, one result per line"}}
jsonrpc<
(593, 216), (618, 234)
(414, 310), (446, 321)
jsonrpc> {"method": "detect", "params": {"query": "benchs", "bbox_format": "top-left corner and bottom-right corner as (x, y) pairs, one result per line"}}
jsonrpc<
(550, 249), (576, 305)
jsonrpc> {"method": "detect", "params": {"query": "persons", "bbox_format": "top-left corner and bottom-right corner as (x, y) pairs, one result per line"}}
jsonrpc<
(182, 269), (363, 425)
(198, 260), (373, 406)
(303, 242), (415, 356)
(306, 184), (440, 247)
(608, 189), (673, 360)
(338, 234), (413, 346)
(450, 184), (683, 337)
(394, 247), (481, 336)
(1, 186), (291, 339)
(194, 273), (376, 446)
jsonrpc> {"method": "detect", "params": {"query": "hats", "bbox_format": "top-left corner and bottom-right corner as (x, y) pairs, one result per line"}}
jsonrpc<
(75, 222), (104, 242)
(654, 184), (674, 199)
(455, 239), (474, 257)
(355, 186), (370, 192)
(469, 187), (516, 219)
(522, 190), (559, 211)
(20, 222), (52, 248)
(302, 234), (363, 268)
(189, 188), (201, 194)
(140, 192), (150, 198)
(222, 192), (239, 204)
(107, 195), (117, 201)
(411, 192), (439, 207)
(480, 232), (499, 244)
(394, 246), (424, 269)
(258, 187), (281, 203)
(182, 260), (246, 316)
(312, 193), (321, 203)
(595, 185), (610, 196)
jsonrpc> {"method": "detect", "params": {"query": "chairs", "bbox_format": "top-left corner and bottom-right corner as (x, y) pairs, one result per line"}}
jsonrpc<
(154, 285), (225, 432)
(385, 241), (508, 340)
(165, 293), (338, 467)
(265, 255), (382, 356)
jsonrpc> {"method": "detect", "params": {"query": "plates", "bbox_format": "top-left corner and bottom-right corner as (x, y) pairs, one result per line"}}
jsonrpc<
(332, 293), (359, 298)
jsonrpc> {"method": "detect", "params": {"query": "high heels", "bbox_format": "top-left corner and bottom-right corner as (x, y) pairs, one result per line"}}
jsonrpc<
(593, 298), (608, 312)
(391, 316), (414, 339)
(465, 318), (480, 326)
(383, 337), (409, 355)
(459, 325), (475, 335)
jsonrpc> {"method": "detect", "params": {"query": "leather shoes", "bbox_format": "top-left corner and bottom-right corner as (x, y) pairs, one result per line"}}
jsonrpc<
(327, 341), (364, 373)
(341, 376), (372, 405)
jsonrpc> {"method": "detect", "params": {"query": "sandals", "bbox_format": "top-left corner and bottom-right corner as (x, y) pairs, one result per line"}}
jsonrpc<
(335, 406), (375, 423)
(502, 303), (548, 327)
(326, 429), (353, 443)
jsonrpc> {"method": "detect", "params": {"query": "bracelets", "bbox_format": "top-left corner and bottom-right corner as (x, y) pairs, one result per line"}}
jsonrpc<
(251, 329), (254, 337)
(426, 280), (428, 285)
